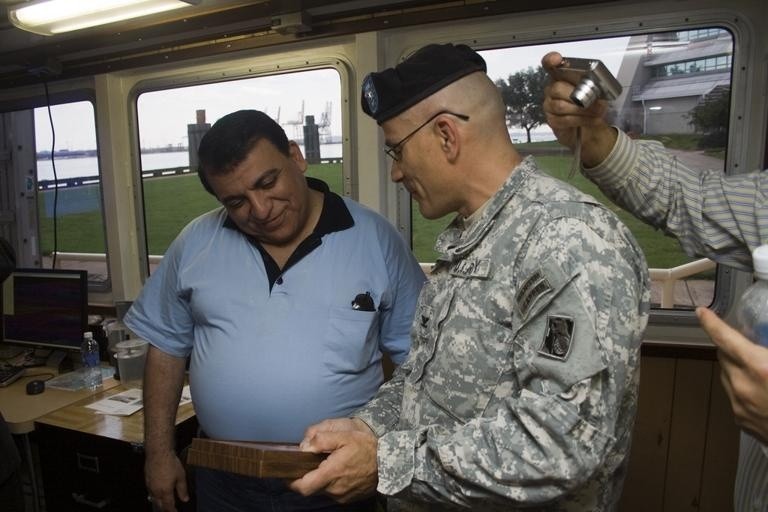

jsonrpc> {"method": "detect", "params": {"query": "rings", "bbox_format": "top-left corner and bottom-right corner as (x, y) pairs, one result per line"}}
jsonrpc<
(147, 496), (152, 502)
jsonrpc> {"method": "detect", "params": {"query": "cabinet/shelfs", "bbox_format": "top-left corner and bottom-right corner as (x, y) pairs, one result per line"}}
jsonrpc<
(34, 375), (200, 512)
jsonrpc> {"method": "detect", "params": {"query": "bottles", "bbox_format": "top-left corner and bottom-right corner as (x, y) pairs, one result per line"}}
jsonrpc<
(734, 244), (768, 460)
(80, 332), (103, 392)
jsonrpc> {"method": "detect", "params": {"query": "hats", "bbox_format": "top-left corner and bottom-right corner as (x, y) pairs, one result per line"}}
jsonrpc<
(361, 42), (488, 125)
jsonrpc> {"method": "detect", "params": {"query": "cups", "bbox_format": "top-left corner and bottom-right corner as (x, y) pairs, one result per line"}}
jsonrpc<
(113, 340), (150, 389)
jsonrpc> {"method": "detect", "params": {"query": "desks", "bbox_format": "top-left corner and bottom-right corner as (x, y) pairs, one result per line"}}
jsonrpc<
(0, 367), (120, 512)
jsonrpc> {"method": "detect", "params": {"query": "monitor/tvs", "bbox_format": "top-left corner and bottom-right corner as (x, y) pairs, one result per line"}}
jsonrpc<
(0, 269), (88, 366)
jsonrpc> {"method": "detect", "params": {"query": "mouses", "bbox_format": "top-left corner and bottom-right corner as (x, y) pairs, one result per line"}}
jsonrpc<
(27, 380), (45, 395)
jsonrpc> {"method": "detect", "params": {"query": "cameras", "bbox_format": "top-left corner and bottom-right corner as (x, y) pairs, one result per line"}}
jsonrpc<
(549, 55), (622, 109)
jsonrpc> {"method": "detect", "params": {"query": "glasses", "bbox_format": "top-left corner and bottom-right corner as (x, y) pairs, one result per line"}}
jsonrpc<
(382, 110), (469, 162)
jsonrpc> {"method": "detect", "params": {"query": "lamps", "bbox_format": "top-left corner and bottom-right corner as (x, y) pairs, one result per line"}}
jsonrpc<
(7, 0), (203, 37)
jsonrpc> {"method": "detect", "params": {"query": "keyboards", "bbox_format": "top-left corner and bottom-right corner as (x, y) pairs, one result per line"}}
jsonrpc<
(0, 361), (25, 387)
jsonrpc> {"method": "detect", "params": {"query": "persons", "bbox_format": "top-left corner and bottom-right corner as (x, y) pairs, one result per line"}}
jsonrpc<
(121, 110), (430, 512)
(543, 46), (768, 511)
(282, 42), (652, 512)
